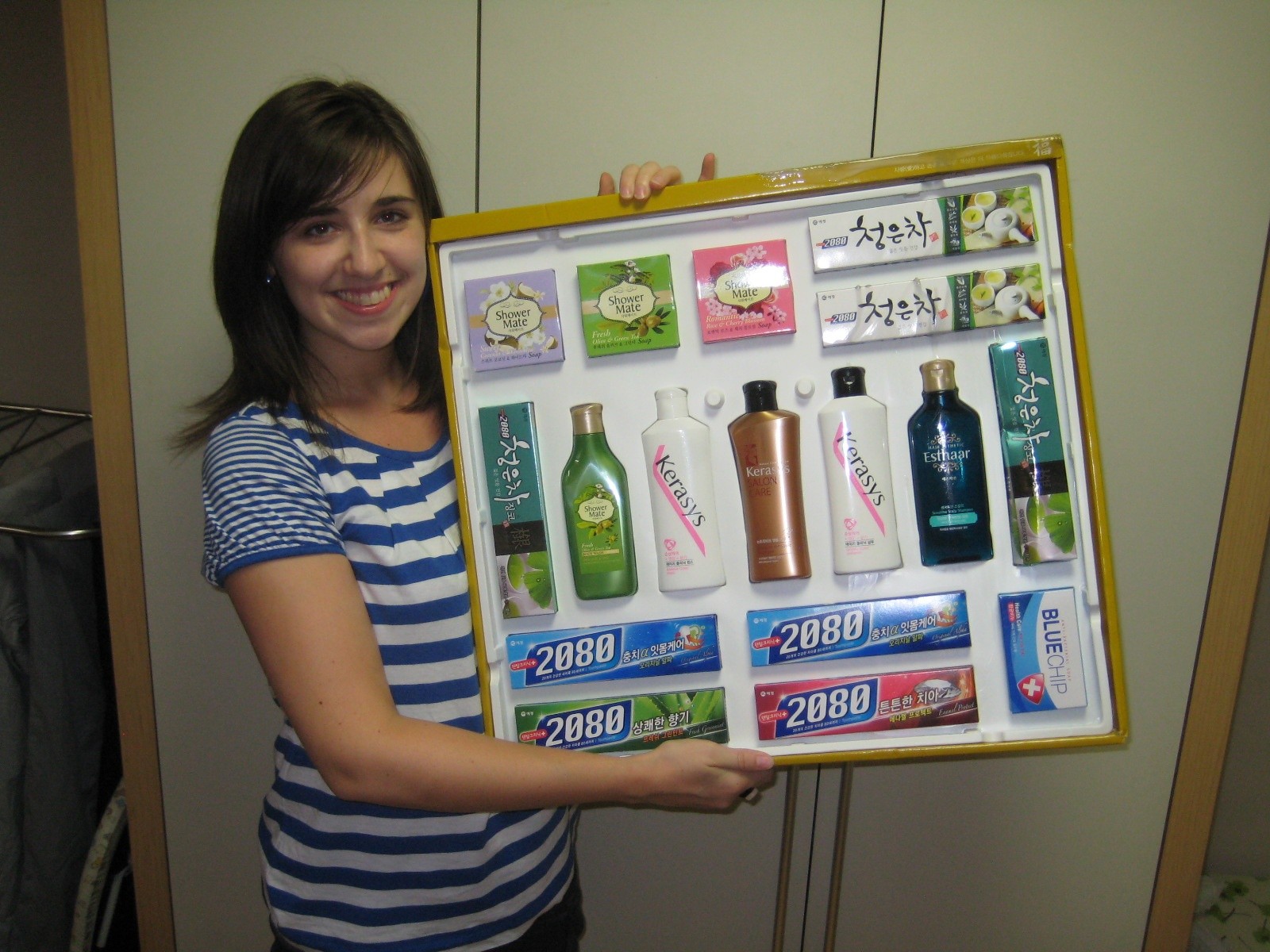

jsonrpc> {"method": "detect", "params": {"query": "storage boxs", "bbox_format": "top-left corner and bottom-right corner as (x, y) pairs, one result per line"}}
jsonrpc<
(427, 134), (1130, 765)
(505, 614), (722, 690)
(575, 255), (680, 357)
(755, 666), (979, 740)
(514, 687), (729, 753)
(817, 258), (1046, 347)
(748, 591), (972, 668)
(478, 401), (559, 621)
(999, 589), (1089, 714)
(988, 337), (1077, 567)
(463, 268), (566, 372)
(807, 186), (1037, 273)
(692, 239), (797, 344)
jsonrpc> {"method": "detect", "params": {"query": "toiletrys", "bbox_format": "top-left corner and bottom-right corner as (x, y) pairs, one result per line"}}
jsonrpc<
(816, 260), (1047, 350)
(727, 380), (812, 584)
(512, 686), (731, 754)
(806, 187), (1041, 274)
(478, 400), (559, 620)
(504, 609), (727, 687)
(987, 336), (1078, 567)
(642, 385), (729, 593)
(560, 402), (639, 602)
(745, 587), (973, 670)
(818, 366), (906, 577)
(753, 666), (981, 742)
(903, 356), (994, 570)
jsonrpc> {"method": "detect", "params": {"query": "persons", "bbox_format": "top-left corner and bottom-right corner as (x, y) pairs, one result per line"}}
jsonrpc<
(170, 78), (778, 952)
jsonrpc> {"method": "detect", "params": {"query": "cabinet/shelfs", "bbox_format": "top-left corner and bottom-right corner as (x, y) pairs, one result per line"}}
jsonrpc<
(58, 1), (1270, 952)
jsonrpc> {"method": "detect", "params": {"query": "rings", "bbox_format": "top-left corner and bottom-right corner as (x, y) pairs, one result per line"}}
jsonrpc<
(739, 785), (759, 801)
